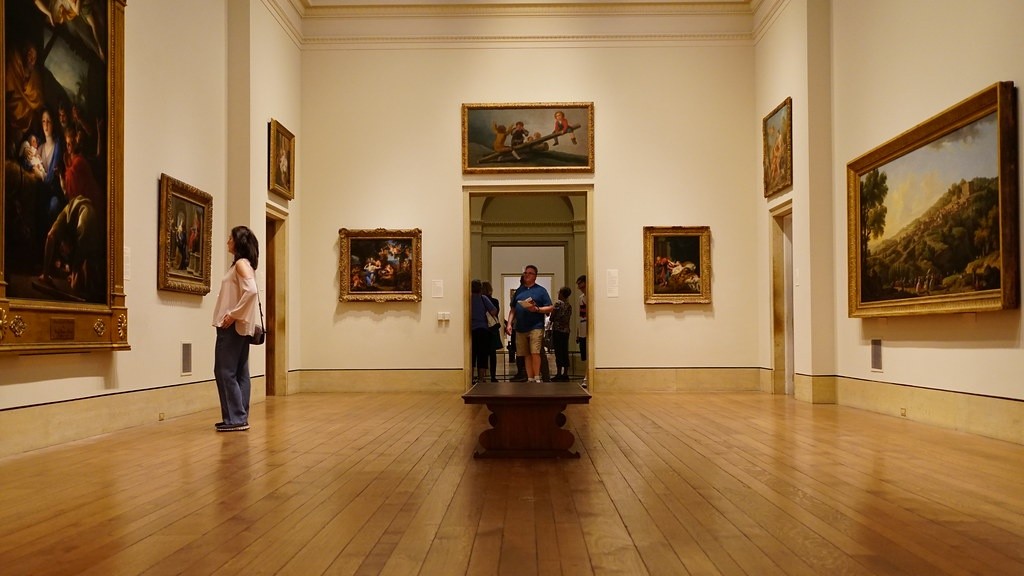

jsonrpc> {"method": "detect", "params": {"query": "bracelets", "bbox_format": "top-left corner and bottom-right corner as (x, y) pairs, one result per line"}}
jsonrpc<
(536, 307), (540, 313)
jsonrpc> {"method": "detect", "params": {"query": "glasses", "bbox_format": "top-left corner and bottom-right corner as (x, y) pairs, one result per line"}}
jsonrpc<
(523, 271), (535, 276)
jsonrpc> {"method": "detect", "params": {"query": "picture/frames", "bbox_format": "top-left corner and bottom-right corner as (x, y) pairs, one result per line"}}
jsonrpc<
(461, 102), (596, 174)
(338, 228), (423, 302)
(0, 0), (131, 353)
(643, 225), (712, 304)
(762, 97), (792, 198)
(158, 172), (213, 296)
(269, 118), (295, 201)
(846, 80), (1018, 317)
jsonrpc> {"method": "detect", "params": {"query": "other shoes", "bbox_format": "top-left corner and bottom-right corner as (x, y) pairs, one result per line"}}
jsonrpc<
(474, 374), (498, 384)
(214, 422), (226, 428)
(216, 422), (250, 431)
(510, 374), (526, 382)
(551, 374), (569, 382)
(581, 379), (587, 387)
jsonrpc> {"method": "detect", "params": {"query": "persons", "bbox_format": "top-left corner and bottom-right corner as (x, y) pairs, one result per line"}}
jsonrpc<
(576, 275), (588, 388)
(212, 226), (259, 430)
(480, 282), (501, 382)
(505, 265), (553, 384)
(471, 279), (499, 386)
(510, 273), (549, 382)
(550, 287), (572, 382)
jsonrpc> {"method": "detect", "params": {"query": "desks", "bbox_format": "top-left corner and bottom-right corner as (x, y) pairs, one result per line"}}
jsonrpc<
(461, 382), (592, 459)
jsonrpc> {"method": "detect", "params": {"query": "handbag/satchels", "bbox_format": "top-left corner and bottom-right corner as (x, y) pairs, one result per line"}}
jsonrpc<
(248, 324), (265, 344)
(480, 295), (501, 330)
